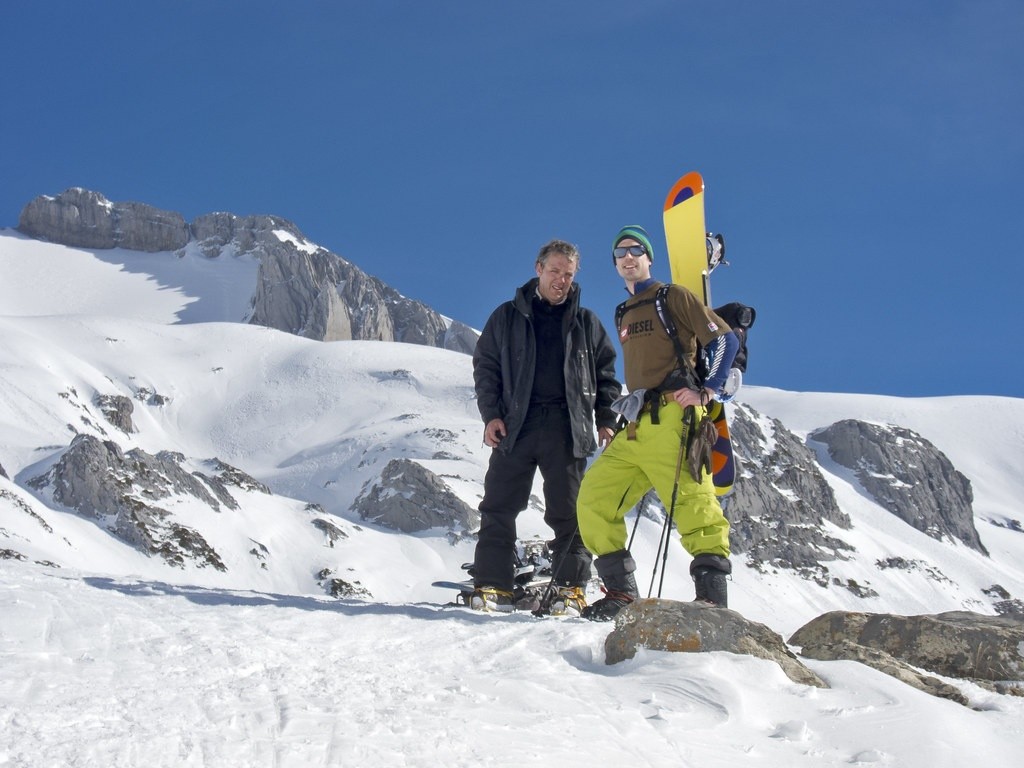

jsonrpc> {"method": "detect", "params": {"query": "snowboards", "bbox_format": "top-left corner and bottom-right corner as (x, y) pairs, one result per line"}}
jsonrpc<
(661, 170), (738, 498)
(429, 557), (603, 595)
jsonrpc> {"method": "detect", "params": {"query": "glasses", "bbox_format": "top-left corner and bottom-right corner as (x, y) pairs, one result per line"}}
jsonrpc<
(613, 245), (647, 258)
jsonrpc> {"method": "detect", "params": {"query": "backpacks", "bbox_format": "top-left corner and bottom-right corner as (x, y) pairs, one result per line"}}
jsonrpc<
(616, 285), (755, 403)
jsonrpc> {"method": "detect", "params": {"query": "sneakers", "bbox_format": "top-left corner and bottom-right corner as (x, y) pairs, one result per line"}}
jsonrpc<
(550, 585), (586, 617)
(468, 586), (514, 612)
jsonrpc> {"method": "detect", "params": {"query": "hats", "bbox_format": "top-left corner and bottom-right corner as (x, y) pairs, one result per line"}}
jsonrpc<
(612, 225), (654, 265)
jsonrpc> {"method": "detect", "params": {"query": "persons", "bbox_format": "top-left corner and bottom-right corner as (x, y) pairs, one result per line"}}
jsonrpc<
(576, 223), (741, 623)
(468, 239), (623, 616)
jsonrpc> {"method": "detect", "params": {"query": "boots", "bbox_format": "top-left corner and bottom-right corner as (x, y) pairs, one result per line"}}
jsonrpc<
(580, 549), (639, 621)
(688, 551), (731, 609)
